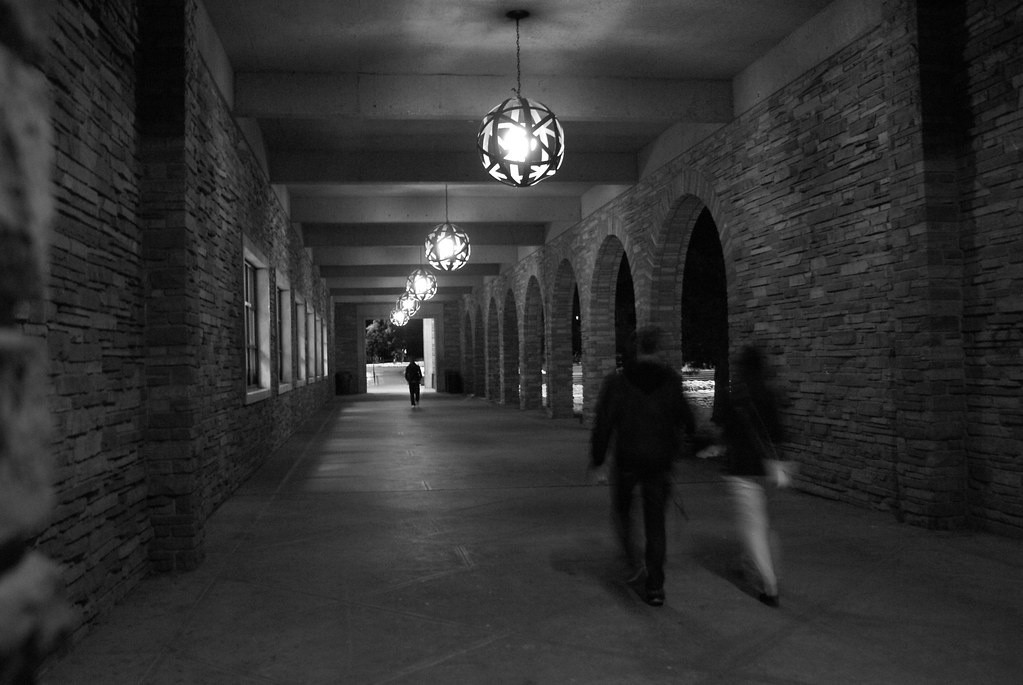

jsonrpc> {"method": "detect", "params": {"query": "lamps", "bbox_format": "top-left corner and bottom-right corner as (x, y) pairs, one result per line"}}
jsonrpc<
(388, 242), (439, 326)
(424, 178), (472, 271)
(474, 11), (566, 188)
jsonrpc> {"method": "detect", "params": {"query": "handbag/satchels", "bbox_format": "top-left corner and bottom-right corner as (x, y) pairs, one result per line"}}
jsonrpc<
(767, 459), (796, 489)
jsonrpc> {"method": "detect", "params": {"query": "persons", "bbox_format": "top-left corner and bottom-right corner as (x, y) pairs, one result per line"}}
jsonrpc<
(710, 367), (789, 609)
(588, 326), (707, 607)
(405, 357), (422, 408)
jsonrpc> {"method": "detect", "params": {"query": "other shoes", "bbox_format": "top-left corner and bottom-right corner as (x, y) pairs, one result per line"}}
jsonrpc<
(627, 564), (644, 586)
(647, 589), (665, 604)
(759, 592), (779, 608)
